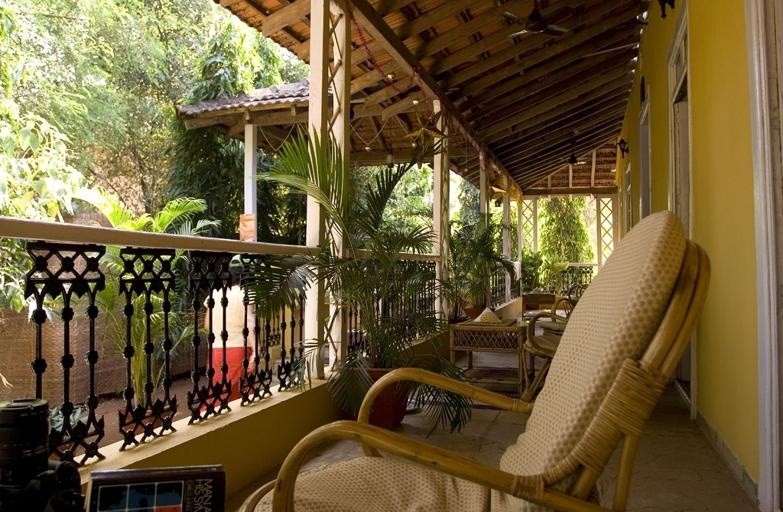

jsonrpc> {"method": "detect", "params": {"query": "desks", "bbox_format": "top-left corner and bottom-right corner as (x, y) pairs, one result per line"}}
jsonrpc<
(449, 318), (536, 397)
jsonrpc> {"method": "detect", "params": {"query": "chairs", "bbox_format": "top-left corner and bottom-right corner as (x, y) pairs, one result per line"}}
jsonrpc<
(239, 210), (711, 511)
(520, 283), (588, 402)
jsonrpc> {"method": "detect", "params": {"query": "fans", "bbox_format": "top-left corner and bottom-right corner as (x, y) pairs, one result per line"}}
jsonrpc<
(497, 1), (573, 39)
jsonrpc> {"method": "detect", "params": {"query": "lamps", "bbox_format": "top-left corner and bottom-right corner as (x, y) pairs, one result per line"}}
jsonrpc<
(615, 138), (629, 159)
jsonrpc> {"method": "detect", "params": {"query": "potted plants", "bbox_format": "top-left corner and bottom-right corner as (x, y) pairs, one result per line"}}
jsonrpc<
(67, 177), (223, 438)
(447, 220), (515, 320)
(259, 121), (473, 439)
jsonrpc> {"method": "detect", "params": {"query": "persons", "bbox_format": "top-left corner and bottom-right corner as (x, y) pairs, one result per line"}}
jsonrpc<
(204, 254), (260, 410)
